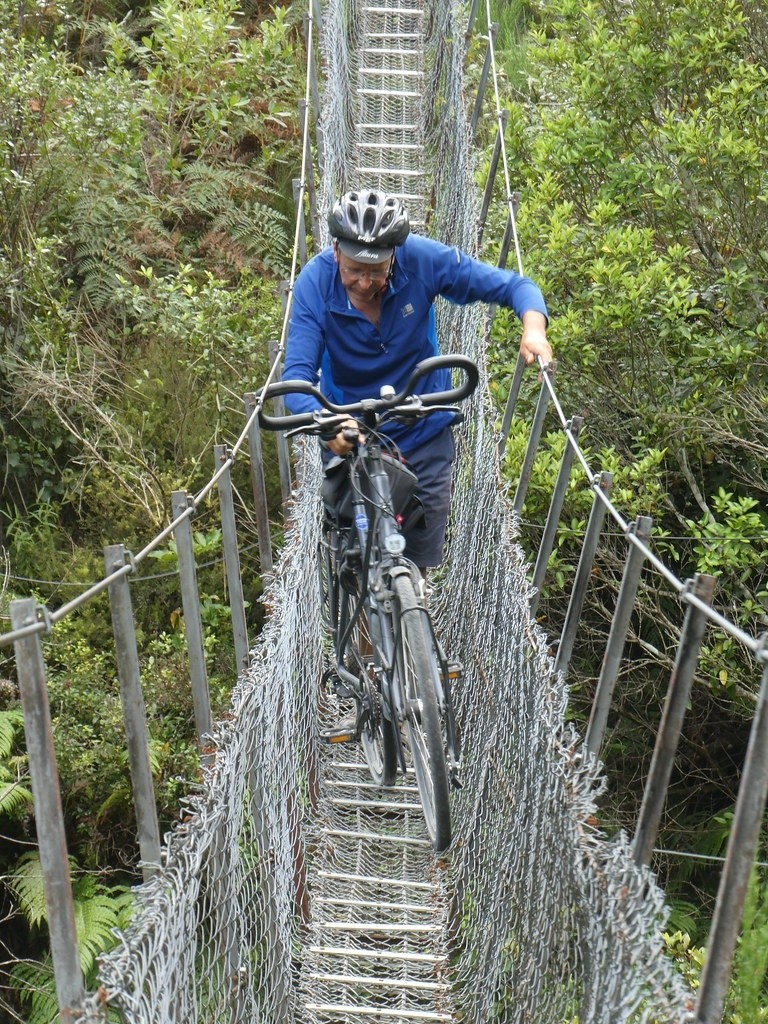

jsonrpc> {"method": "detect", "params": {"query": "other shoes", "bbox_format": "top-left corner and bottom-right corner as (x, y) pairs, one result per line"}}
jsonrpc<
(337, 665), (360, 697)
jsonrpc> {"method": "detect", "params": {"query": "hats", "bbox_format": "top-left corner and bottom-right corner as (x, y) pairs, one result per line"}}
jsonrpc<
(339, 238), (393, 264)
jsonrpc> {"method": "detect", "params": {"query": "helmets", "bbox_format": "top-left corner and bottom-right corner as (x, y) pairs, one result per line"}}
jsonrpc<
(328, 189), (410, 246)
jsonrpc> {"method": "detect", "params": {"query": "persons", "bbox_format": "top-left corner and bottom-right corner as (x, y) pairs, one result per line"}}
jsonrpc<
(282, 190), (553, 769)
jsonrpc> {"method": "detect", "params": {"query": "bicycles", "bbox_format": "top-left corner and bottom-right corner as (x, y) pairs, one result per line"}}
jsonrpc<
(255, 353), (480, 853)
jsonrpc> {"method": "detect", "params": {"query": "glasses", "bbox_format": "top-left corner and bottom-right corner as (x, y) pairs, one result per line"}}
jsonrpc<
(340, 252), (395, 280)
